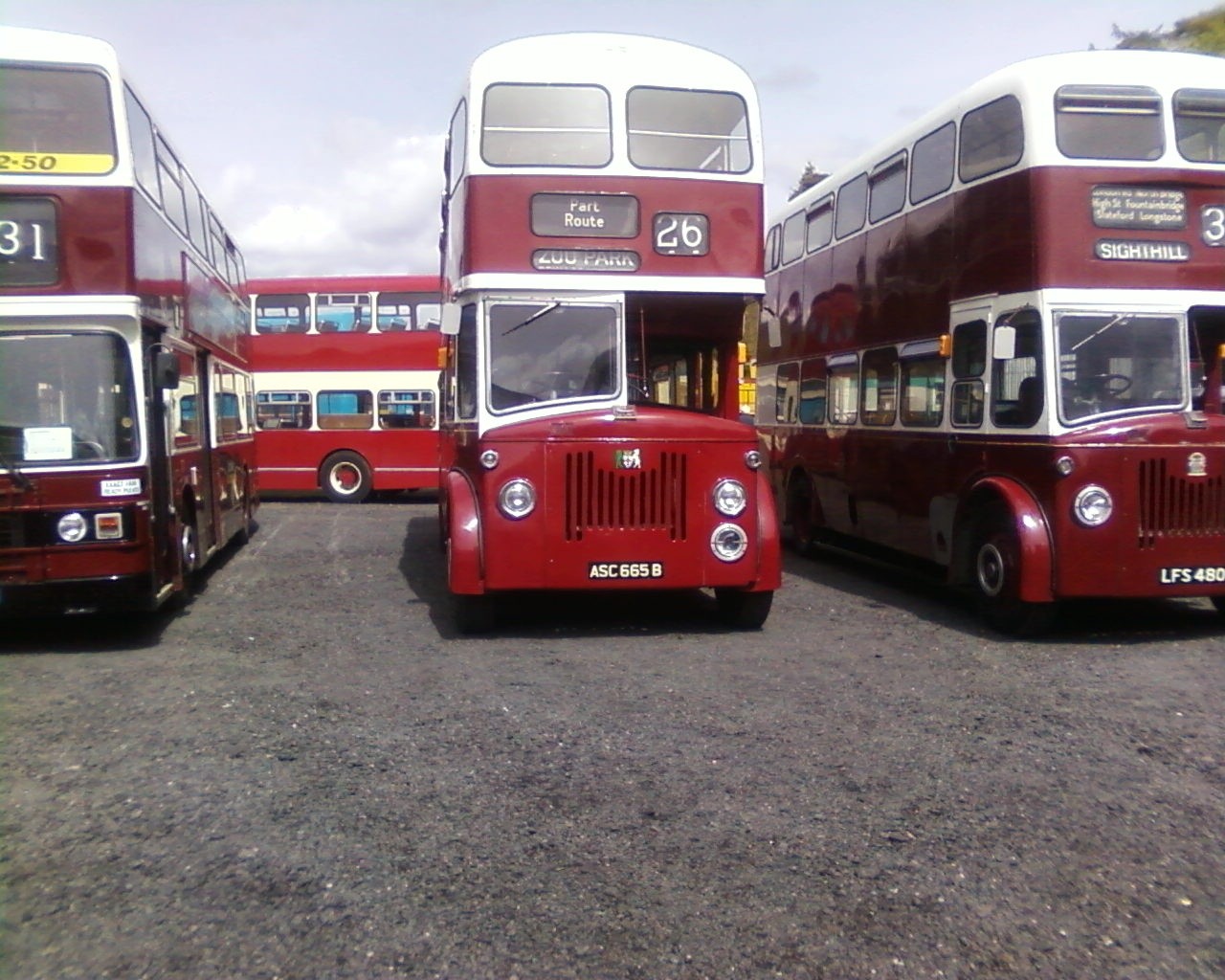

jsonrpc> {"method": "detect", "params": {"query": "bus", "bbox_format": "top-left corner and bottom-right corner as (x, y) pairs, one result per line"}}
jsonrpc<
(756, 50), (1225, 640)
(434, 28), (788, 631)
(1, 29), (257, 639)
(238, 274), (450, 501)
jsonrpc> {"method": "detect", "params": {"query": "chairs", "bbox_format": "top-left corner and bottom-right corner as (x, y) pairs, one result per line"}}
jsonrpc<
(282, 316), (441, 331)
(263, 416), (282, 429)
